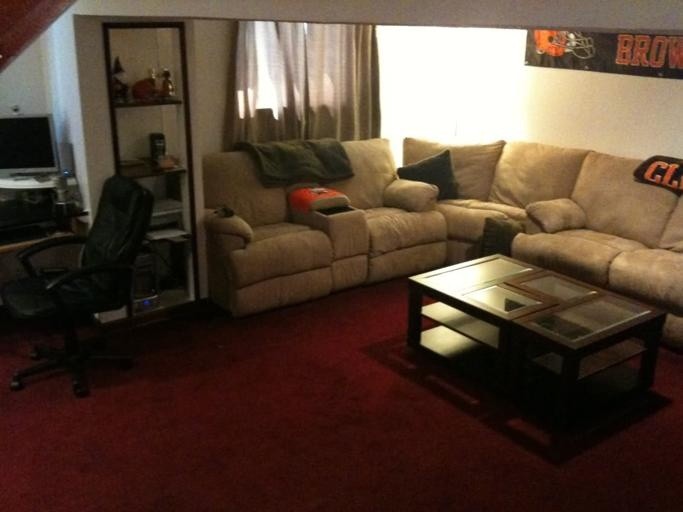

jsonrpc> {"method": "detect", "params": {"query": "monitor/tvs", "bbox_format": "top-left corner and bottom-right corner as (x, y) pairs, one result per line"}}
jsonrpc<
(1, 113), (60, 174)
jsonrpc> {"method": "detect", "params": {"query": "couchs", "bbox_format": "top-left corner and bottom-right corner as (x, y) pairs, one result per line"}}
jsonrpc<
(210, 136), (447, 316)
(404, 134), (683, 349)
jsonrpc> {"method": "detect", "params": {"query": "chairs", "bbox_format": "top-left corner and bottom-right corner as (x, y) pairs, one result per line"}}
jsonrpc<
(4, 174), (152, 396)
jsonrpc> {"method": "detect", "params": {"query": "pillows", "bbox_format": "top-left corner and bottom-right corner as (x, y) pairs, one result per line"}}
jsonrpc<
(397, 150), (455, 201)
(384, 178), (437, 214)
(530, 197), (586, 230)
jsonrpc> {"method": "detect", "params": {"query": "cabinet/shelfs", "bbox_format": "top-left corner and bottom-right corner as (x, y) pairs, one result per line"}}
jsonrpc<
(103, 20), (205, 334)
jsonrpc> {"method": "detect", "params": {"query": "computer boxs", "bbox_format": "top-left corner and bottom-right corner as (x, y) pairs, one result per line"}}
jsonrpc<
(136, 240), (161, 315)
